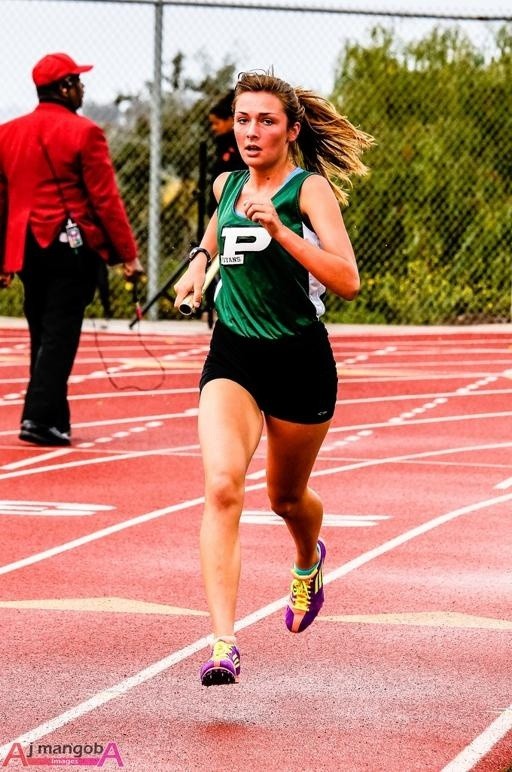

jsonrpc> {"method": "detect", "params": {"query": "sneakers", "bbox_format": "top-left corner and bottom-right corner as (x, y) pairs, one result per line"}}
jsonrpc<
(285, 537), (326, 633)
(200, 639), (241, 686)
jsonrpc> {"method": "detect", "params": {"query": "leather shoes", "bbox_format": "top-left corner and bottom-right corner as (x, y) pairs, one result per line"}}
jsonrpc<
(20, 419), (70, 446)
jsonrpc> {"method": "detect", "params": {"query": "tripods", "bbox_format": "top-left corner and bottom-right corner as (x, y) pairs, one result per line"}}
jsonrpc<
(130, 138), (217, 334)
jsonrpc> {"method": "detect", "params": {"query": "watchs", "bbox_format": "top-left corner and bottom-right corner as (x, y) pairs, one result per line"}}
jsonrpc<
(187, 246), (212, 272)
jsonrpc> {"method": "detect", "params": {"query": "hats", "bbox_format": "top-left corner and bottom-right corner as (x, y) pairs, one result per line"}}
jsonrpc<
(32, 53), (94, 88)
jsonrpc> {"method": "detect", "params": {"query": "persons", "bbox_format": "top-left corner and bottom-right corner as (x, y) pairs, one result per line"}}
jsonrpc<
(0, 50), (146, 451)
(199, 93), (251, 170)
(170, 72), (360, 691)
